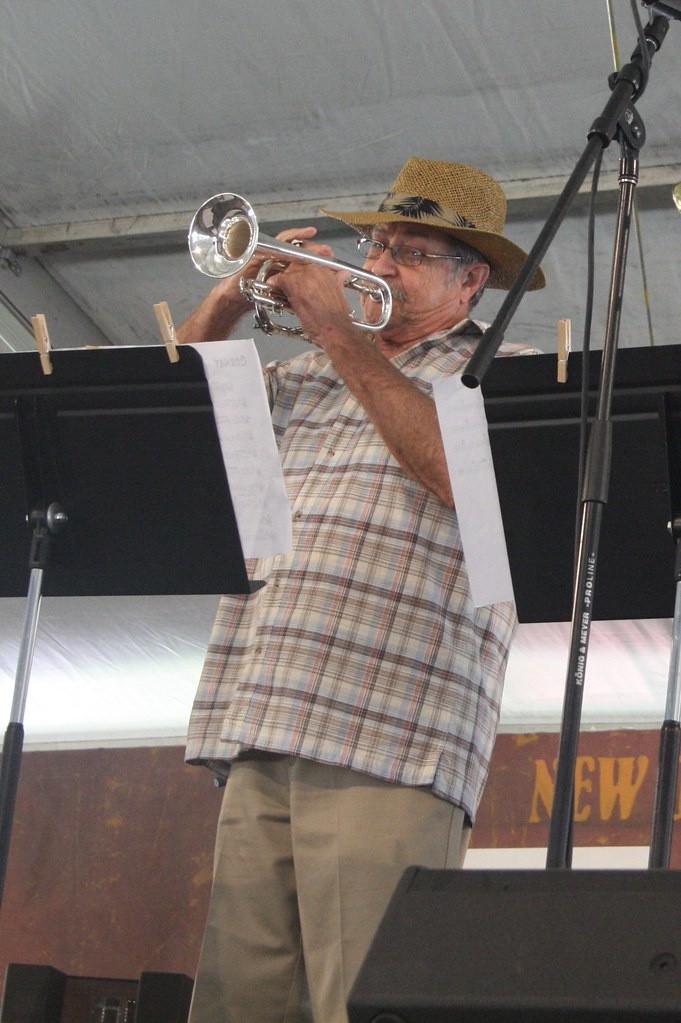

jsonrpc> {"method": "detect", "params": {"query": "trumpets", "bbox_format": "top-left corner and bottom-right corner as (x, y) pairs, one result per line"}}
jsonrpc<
(186, 191), (392, 345)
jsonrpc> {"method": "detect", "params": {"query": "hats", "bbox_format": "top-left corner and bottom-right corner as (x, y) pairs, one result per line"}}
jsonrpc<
(320, 157), (547, 292)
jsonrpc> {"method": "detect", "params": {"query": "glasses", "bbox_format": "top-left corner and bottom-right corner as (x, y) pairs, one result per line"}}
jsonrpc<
(356, 237), (465, 267)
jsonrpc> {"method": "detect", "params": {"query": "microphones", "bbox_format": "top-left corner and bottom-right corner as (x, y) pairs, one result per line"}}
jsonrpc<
(640, 0), (681, 22)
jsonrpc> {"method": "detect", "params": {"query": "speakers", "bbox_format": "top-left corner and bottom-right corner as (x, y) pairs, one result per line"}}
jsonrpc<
(1, 963), (194, 1023)
(344, 863), (681, 1023)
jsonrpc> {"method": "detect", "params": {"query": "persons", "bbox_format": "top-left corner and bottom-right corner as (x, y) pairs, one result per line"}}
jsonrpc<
(171, 157), (547, 1023)
